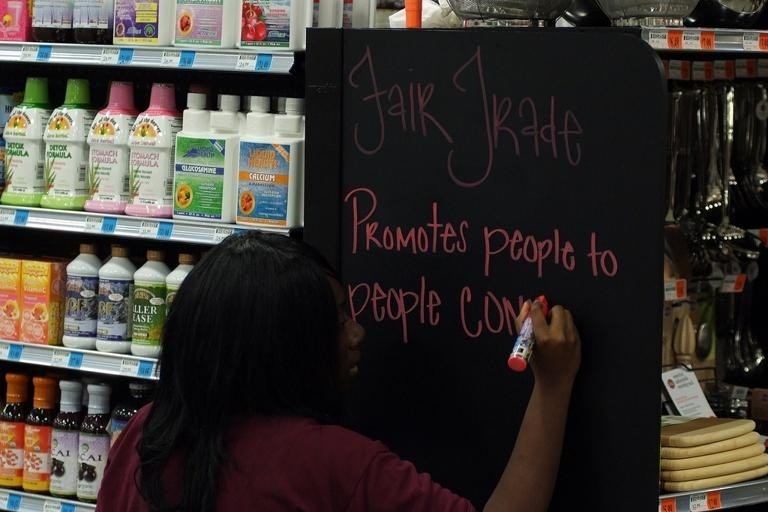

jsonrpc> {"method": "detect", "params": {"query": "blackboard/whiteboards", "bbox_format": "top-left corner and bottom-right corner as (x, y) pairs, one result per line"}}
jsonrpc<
(305, 26), (669, 512)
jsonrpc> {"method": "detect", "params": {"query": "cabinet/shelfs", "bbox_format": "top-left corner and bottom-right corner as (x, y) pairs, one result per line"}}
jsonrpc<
(643, 26), (767, 512)
(0, 40), (306, 512)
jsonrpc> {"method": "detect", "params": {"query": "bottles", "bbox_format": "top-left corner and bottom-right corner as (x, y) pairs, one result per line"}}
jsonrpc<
(0, 375), (30, 487)
(130, 252), (171, 357)
(24, 378), (55, 492)
(62, 245), (102, 350)
(76, 385), (116, 504)
(164, 256), (197, 319)
(109, 385), (150, 451)
(48, 380), (85, 496)
(94, 249), (137, 351)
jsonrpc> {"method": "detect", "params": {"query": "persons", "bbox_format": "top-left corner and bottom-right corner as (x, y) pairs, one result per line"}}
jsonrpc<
(97, 230), (583, 511)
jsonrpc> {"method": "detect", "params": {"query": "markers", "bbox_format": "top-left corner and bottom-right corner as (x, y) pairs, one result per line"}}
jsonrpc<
(508, 295), (548, 372)
